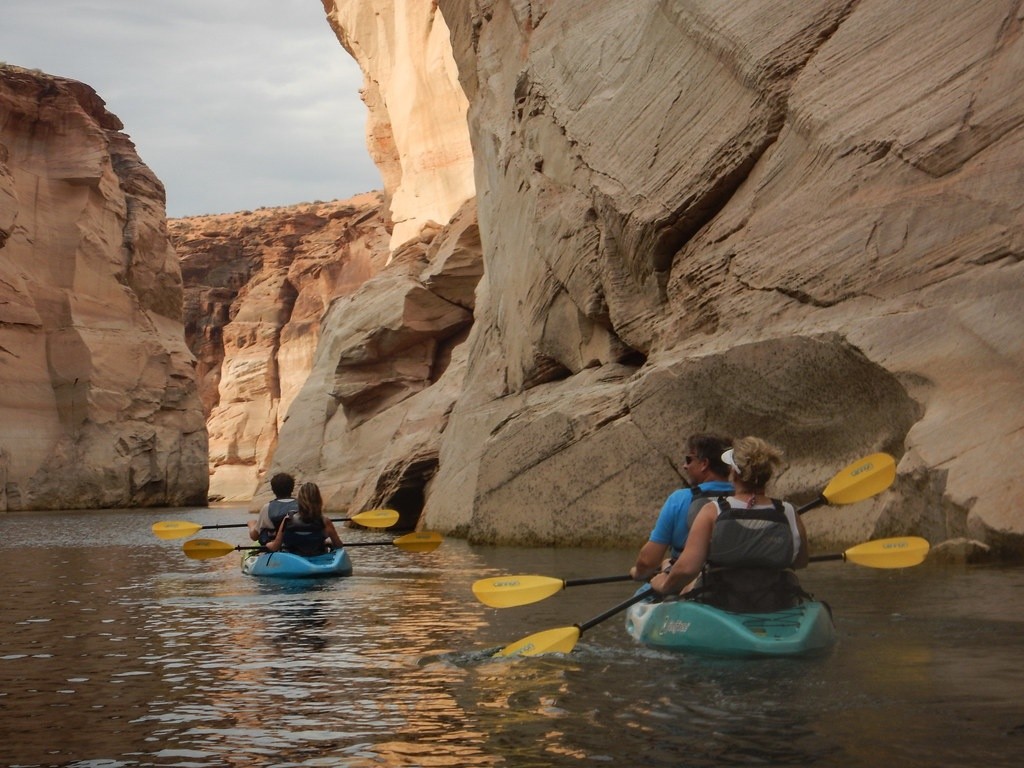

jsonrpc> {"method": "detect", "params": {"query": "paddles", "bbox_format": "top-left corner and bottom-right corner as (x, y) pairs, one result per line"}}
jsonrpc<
(468, 533), (933, 611)
(179, 530), (445, 561)
(490, 451), (899, 662)
(151, 509), (401, 542)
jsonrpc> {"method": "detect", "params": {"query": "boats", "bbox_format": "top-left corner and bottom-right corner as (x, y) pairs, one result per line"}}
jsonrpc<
(624, 567), (839, 660)
(239, 540), (353, 578)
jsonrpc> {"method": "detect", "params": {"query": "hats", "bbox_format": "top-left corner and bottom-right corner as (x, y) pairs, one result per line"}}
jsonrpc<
(721, 448), (741, 474)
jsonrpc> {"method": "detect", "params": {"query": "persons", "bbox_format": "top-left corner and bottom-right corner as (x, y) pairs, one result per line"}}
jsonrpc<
(247, 473), (298, 547)
(627, 431), (735, 581)
(266, 481), (344, 557)
(647, 436), (810, 613)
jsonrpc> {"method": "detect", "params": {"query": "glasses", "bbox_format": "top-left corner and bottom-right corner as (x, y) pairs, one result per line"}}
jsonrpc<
(685, 455), (698, 464)
